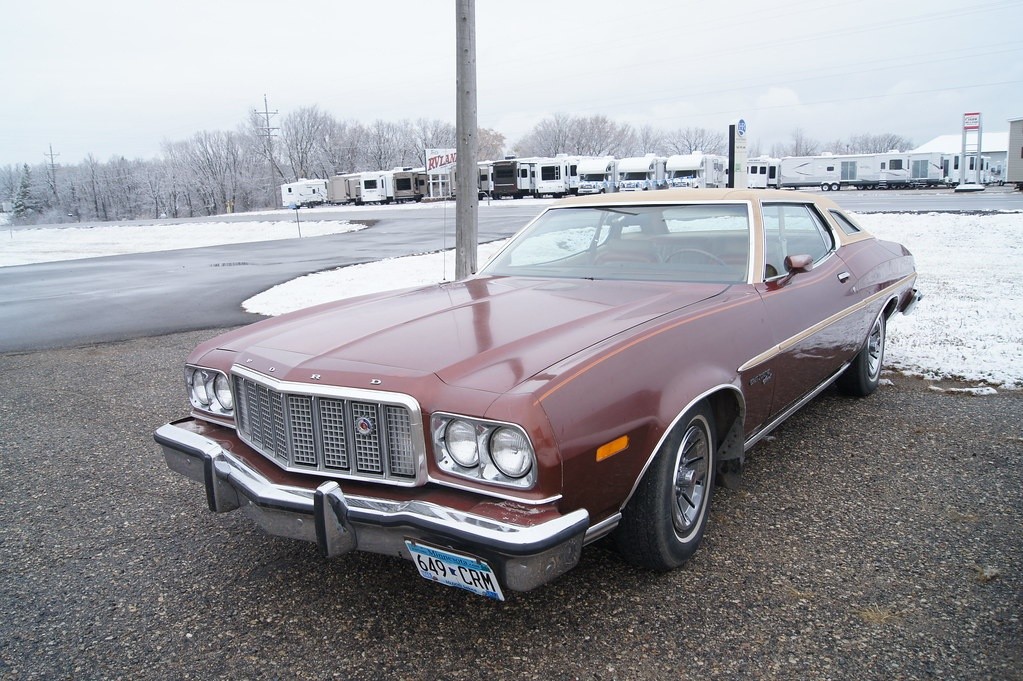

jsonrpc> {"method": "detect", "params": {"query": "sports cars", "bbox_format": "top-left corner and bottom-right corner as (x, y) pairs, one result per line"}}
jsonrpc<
(150, 189), (924, 606)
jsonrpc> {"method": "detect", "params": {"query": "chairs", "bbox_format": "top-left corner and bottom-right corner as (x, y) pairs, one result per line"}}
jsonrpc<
(712, 237), (785, 274)
(591, 238), (664, 275)
